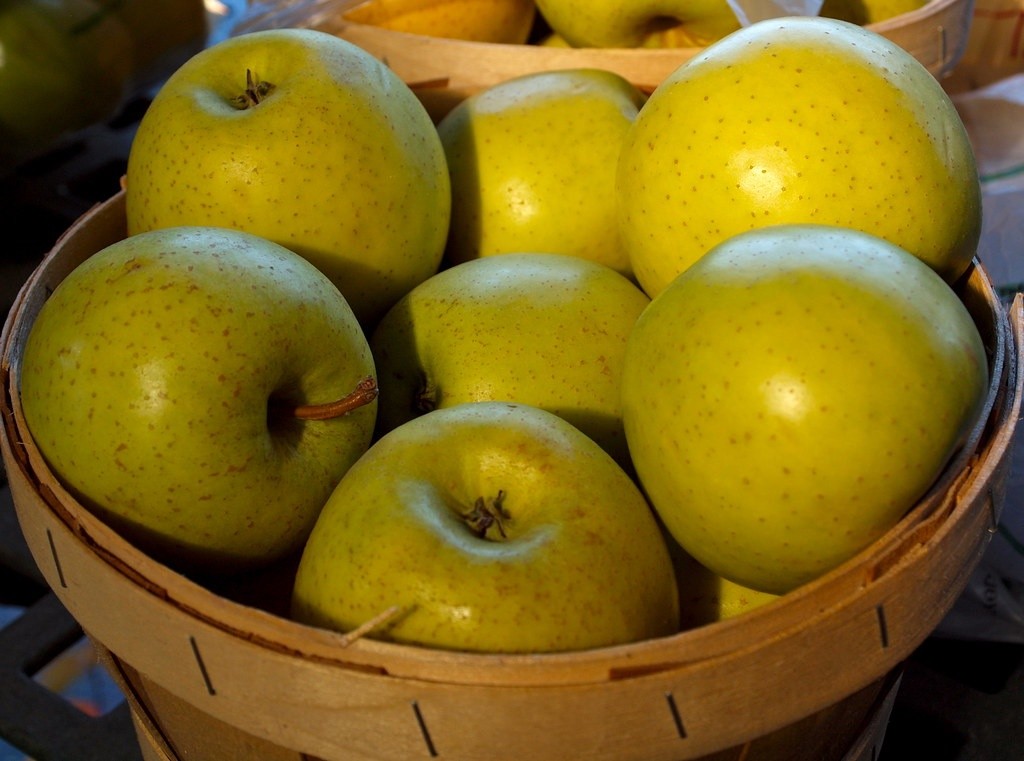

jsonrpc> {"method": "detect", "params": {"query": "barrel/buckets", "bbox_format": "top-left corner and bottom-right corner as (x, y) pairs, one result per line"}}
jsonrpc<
(200, 0), (972, 103)
(0, 169), (1024, 759)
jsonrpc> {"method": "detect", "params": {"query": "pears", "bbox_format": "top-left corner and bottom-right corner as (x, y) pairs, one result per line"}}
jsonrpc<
(17, 0), (986, 658)
